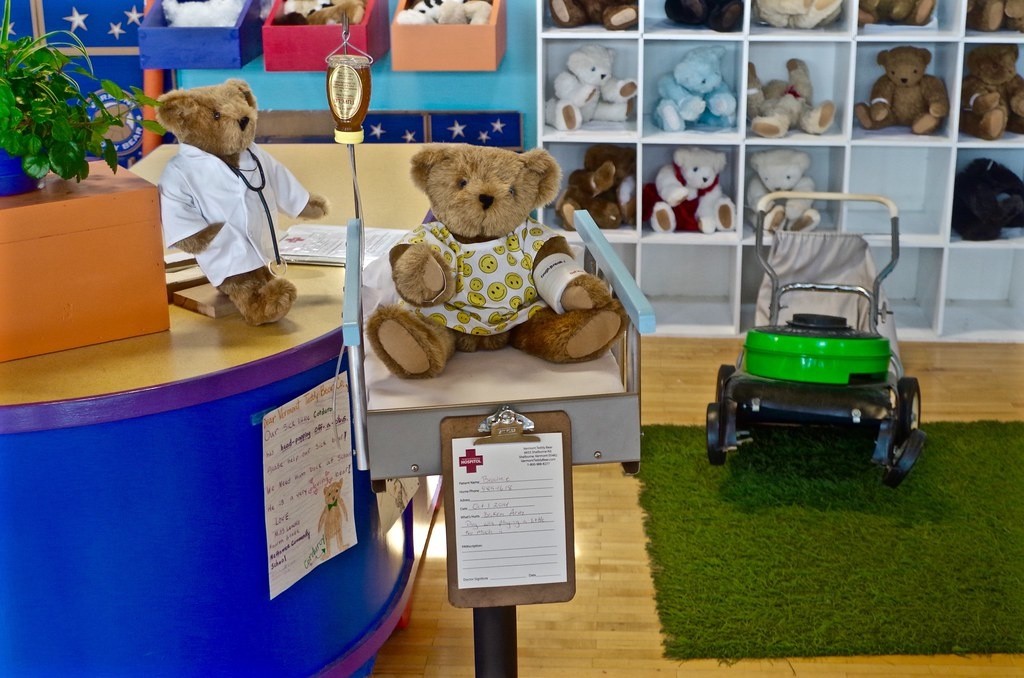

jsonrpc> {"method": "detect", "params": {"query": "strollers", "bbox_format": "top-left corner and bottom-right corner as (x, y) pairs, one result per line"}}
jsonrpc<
(705, 191), (926, 489)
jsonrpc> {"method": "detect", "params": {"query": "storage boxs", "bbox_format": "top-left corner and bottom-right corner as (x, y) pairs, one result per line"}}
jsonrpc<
(139, 0), (507, 71)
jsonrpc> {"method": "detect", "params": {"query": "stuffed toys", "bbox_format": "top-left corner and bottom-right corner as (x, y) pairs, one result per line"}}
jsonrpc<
(160, 0), (247, 27)
(556, 143), (636, 230)
(743, 150), (822, 234)
(641, 148), (735, 235)
(549, 1), (1023, 32)
(855, 47), (949, 135)
(366, 143), (631, 381)
(652, 44), (737, 133)
(959, 43), (1024, 140)
(544, 43), (639, 130)
(154, 78), (328, 326)
(271, 0), (493, 26)
(748, 58), (836, 139)
(951, 158), (1023, 242)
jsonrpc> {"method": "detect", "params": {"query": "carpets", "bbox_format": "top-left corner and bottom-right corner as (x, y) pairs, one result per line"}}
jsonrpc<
(633, 419), (1024, 660)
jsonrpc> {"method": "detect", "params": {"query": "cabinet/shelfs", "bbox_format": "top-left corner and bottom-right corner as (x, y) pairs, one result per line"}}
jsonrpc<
(535, 0), (1024, 344)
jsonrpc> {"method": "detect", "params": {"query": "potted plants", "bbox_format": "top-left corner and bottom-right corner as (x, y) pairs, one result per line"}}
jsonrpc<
(0, 0), (168, 199)
